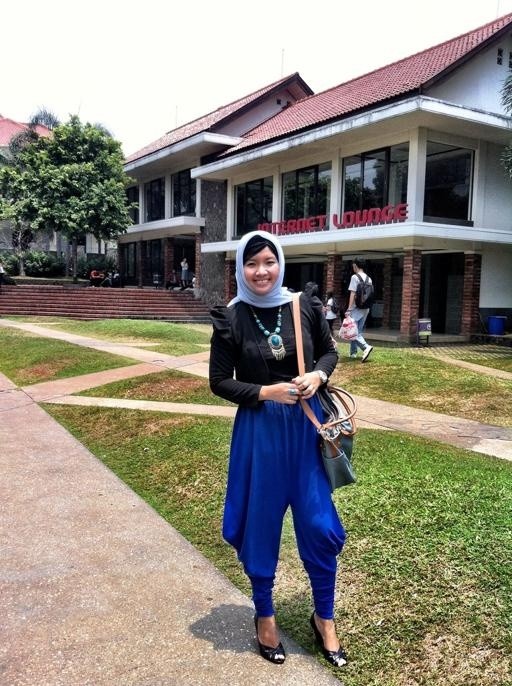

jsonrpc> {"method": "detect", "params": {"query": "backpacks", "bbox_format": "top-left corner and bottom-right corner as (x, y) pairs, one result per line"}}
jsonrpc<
(331, 296), (342, 313)
(354, 273), (374, 309)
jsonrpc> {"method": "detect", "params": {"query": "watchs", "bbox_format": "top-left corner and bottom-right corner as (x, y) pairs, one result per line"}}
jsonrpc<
(316, 369), (327, 385)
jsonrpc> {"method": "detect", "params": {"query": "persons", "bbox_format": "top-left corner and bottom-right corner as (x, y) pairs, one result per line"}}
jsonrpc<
(345, 256), (376, 363)
(305, 281), (323, 313)
(203, 230), (355, 666)
(90, 267), (119, 288)
(181, 258), (189, 287)
(322, 292), (338, 335)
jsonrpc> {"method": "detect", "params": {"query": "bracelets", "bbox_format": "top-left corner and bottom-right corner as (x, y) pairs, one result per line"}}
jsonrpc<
(347, 309), (352, 311)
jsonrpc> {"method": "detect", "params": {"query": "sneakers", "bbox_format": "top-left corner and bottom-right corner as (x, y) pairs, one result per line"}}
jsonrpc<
(350, 354), (357, 358)
(362, 346), (373, 361)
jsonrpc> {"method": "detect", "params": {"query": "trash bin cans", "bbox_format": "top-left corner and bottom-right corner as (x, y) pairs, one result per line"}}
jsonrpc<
(487, 316), (509, 335)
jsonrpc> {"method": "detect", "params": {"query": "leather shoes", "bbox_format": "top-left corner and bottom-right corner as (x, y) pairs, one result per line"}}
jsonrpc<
(310, 610), (349, 667)
(254, 611), (285, 663)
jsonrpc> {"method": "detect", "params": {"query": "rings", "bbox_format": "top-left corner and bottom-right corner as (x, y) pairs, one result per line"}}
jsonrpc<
(308, 384), (313, 388)
(288, 388), (295, 394)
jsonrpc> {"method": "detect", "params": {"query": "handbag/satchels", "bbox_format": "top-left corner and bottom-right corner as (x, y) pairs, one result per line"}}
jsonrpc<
(310, 384), (357, 489)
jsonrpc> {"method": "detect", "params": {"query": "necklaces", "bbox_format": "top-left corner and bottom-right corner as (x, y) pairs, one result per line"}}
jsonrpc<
(250, 299), (287, 360)
